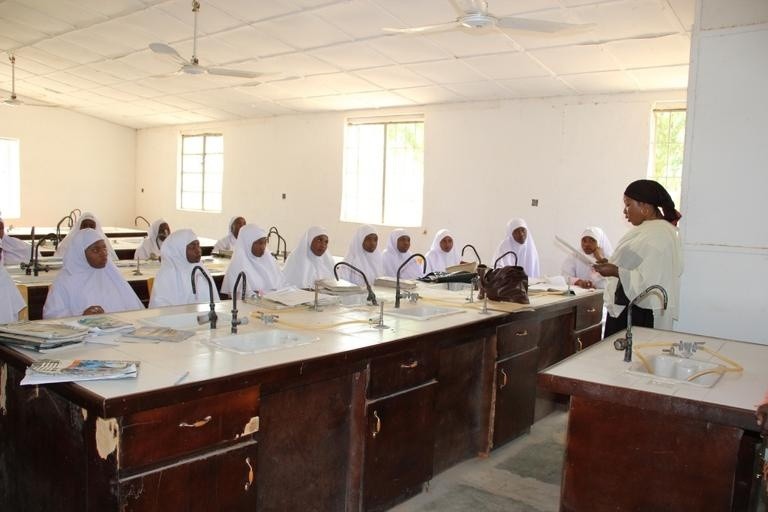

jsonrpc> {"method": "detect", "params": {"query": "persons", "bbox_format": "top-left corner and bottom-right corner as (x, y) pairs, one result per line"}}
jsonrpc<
(212, 215), (246, 252)
(134, 219), (171, 260)
(53, 211), (119, 261)
(492, 218), (540, 278)
(0, 237), (27, 325)
(422, 229), (461, 275)
(593, 179), (681, 339)
(0, 211), (42, 266)
(560, 226), (612, 340)
(339, 225), (385, 287)
(42, 227), (146, 319)
(381, 228), (424, 281)
(148, 228), (220, 308)
(220, 224), (286, 300)
(282, 226), (336, 289)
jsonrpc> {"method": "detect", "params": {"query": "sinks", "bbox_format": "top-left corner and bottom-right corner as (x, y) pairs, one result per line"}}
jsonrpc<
(626, 351), (727, 389)
(336, 294), (389, 309)
(428, 281), (472, 293)
(378, 304), (466, 320)
(137, 311), (231, 332)
(207, 327), (320, 354)
(11, 273), (58, 283)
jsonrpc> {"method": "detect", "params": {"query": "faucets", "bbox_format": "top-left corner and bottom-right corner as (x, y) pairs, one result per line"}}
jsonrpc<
(269, 227), (280, 255)
(34, 233), (58, 274)
(334, 262), (377, 304)
(68, 209), (81, 227)
(69, 211), (76, 227)
(135, 216), (151, 229)
(266, 232), (287, 262)
(230, 272), (246, 332)
(57, 216), (73, 239)
(395, 253), (427, 307)
(191, 266), (217, 330)
(461, 243), (481, 266)
(493, 251), (518, 270)
(625, 286), (668, 362)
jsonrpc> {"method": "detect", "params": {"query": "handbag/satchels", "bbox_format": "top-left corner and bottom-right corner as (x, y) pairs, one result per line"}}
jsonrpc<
(416, 270), (477, 283)
(478, 251), (530, 304)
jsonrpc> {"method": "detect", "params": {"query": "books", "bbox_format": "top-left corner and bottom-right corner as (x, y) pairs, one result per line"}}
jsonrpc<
(19, 359), (140, 385)
(375, 276), (416, 289)
(527, 275), (595, 296)
(0, 314), (197, 354)
(255, 277), (368, 310)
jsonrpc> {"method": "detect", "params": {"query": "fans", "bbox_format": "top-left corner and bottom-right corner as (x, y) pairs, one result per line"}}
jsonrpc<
(127, 1), (263, 82)
(0, 52), (59, 109)
(384, 2), (581, 43)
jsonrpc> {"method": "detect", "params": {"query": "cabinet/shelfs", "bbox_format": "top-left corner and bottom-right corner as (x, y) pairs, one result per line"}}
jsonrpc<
(358, 345), (439, 512)
(570, 296), (603, 355)
(486, 313), (541, 451)
(104, 378), (264, 512)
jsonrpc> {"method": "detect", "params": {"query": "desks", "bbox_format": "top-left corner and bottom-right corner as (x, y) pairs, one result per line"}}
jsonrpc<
(9, 225), (146, 236)
(3, 255), (225, 321)
(19, 236), (217, 257)
(540, 348), (588, 511)
